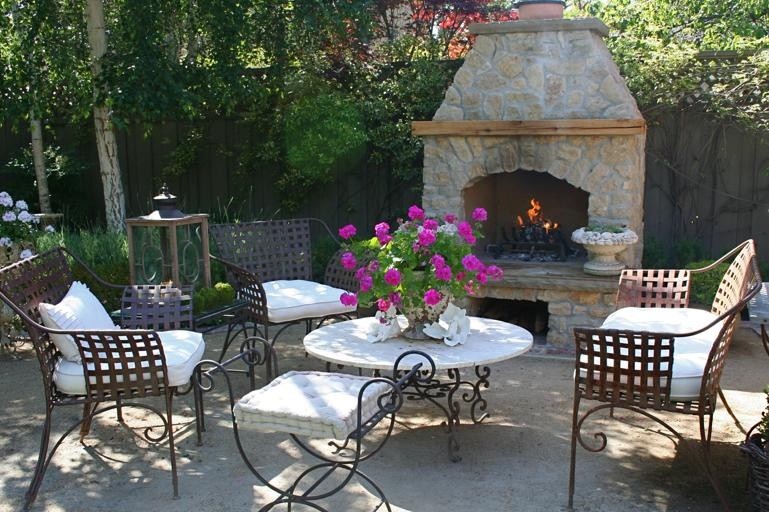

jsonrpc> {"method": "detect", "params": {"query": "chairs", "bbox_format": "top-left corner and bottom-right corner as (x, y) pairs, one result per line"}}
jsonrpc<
(186, 217), (378, 386)
(0, 243), (204, 507)
(565, 239), (763, 512)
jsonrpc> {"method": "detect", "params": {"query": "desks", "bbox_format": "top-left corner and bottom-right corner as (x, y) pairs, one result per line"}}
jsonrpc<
(301, 313), (536, 464)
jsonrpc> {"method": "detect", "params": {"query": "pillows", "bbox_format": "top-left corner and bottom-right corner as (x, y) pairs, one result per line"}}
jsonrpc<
(39, 278), (119, 360)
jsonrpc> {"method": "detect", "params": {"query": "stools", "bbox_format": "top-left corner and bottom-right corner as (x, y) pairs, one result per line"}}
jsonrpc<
(195, 336), (437, 512)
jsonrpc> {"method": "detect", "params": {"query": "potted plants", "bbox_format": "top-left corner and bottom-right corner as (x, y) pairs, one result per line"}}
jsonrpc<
(573, 218), (639, 276)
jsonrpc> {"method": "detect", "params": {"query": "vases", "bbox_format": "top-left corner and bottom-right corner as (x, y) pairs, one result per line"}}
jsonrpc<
(389, 269), (450, 340)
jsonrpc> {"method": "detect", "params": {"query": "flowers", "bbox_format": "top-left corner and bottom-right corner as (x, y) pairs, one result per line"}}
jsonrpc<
(333, 200), (506, 324)
